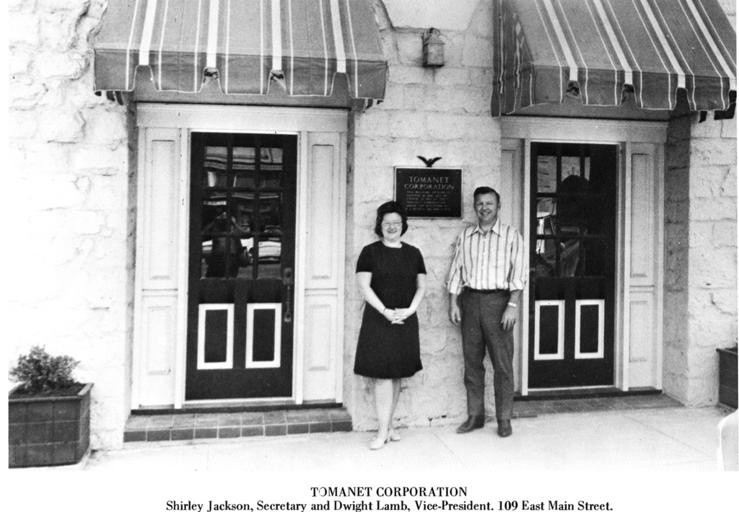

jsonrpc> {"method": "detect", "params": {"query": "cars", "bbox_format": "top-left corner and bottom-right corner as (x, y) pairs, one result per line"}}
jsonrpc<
(202, 228), (282, 263)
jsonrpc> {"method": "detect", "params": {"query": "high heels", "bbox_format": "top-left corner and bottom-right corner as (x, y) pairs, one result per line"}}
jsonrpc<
(370, 430), (401, 450)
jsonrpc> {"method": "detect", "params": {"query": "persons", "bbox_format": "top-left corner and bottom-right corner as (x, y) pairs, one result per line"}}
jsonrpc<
(200, 211), (250, 275)
(351, 198), (431, 451)
(441, 185), (528, 437)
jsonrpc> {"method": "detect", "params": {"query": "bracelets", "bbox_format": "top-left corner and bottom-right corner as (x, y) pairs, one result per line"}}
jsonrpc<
(507, 302), (517, 309)
(383, 307), (386, 315)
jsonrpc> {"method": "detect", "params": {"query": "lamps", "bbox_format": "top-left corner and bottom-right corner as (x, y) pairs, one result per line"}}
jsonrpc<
(422, 27), (445, 67)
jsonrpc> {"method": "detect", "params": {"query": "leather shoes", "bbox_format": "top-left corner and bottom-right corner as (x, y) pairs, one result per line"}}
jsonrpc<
(456, 415), (484, 433)
(497, 418), (512, 436)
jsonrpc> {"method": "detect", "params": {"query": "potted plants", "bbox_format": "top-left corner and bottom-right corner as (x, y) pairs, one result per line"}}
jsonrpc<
(8, 345), (94, 469)
(716, 342), (737, 408)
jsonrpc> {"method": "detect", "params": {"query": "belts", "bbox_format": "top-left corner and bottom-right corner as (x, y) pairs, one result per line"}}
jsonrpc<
(463, 287), (502, 294)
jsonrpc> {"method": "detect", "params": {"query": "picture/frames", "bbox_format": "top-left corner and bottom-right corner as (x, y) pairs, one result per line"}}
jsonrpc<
(394, 165), (465, 220)
